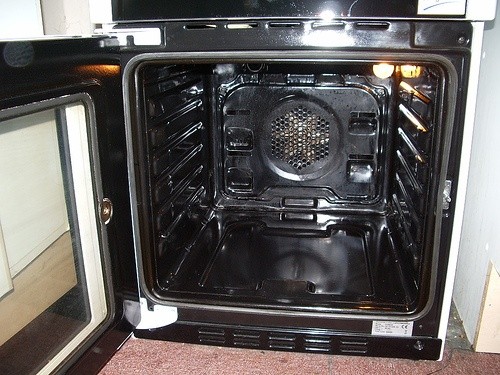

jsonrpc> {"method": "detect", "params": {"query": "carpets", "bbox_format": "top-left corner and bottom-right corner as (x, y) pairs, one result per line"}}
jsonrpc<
(98, 333), (500, 374)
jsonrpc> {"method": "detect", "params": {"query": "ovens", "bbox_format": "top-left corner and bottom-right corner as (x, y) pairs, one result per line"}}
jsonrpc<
(1, 19), (485, 375)
